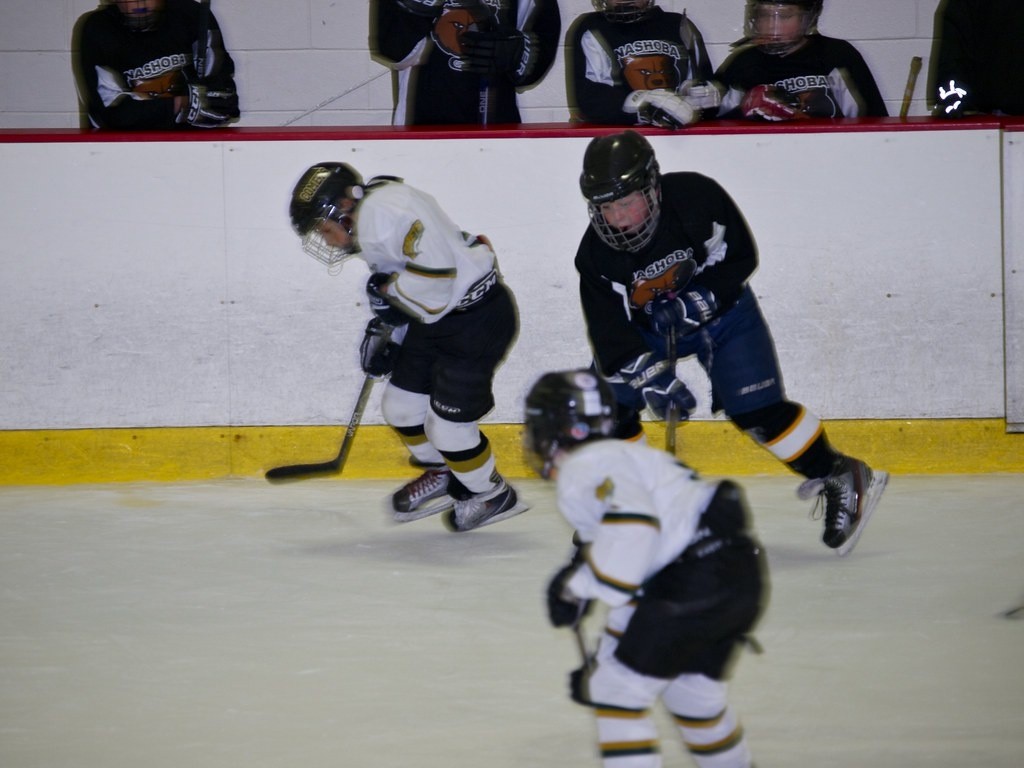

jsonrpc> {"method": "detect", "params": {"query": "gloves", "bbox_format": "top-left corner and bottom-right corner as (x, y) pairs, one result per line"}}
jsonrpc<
(366, 266), (410, 326)
(617, 346), (696, 428)
(457, 27), (530, 78)
(360, 317), (404, 380)
(549, 562), (598, 625)
(651, 280), (732, 341)
(171, 77), (241, 129)
(741, 82), (807, 123)
(570, 656), (598, 705)
(621, 78), (721, 129)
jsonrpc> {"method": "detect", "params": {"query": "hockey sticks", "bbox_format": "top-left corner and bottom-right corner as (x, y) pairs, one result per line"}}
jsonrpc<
(666, 322), (677, 456)
(266, 324), (395, 485)
(573, 627), (590, 662)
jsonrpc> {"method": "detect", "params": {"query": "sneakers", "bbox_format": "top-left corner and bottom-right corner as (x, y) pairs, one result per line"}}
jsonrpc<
(796, 452), (889, 555)
(447, 466), (531, 531)
(391, 456), (456, 521)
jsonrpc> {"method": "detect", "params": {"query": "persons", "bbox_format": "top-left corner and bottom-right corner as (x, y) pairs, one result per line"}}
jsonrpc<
(70, 0), (240, 131)
(574, 130), (887, 553)
(565, 0), (715, 128)
(375, 0), (561, 126)
(525, 369), (763, 768)
(722, 0), (890, 122)
(938, 0), (1024, 119)
(290, 161), (533, 529)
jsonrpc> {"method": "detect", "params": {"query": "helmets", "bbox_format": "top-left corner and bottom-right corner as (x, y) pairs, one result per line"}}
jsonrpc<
(580, 130), (660, 254)
(108, 0), (168, 33)
(744, 1), (823, 57)
(290, 163), (365, 266)
(522, 369), (618, 482)
